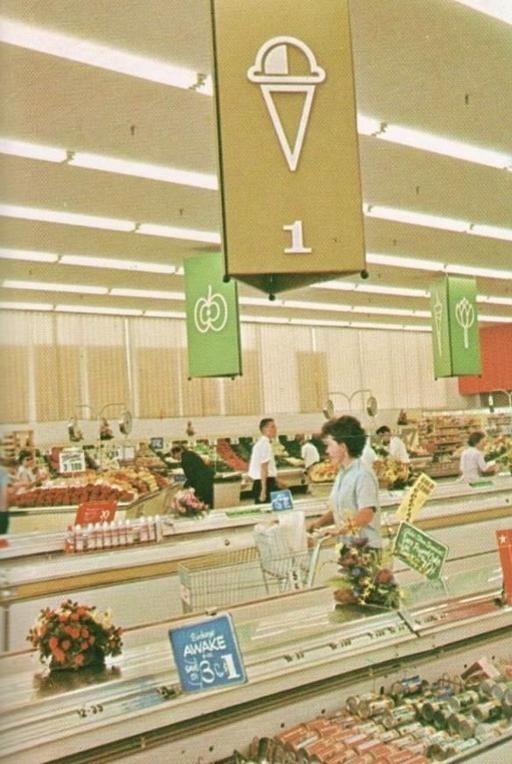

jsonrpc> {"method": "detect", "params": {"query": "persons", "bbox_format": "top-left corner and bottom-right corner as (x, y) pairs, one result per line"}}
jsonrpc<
(375, 425), (411, 463)
(306, 415), (383, 564)
(170, 445), (214, 509)
(459, 432), (496, 482)
(0, 467), (10, 534)
(299, 440), (320, 469)
(247, 418), (281, 504)
(16, 450), (34, 481)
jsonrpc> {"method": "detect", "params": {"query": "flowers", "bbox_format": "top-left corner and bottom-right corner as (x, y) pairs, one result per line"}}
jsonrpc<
(25, 595), (129, 687)
(169, 490), (212, 520)
(332, 535), (404, 614)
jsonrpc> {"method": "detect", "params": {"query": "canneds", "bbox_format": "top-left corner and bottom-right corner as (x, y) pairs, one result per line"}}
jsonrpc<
(250, 675), (512, 764)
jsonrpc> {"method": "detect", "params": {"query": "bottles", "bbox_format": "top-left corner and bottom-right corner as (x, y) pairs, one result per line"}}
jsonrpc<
(64, 514), (164, 553)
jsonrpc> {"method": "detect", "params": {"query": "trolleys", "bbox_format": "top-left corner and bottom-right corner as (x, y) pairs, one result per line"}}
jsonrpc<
(177, 524), (333, 614)
